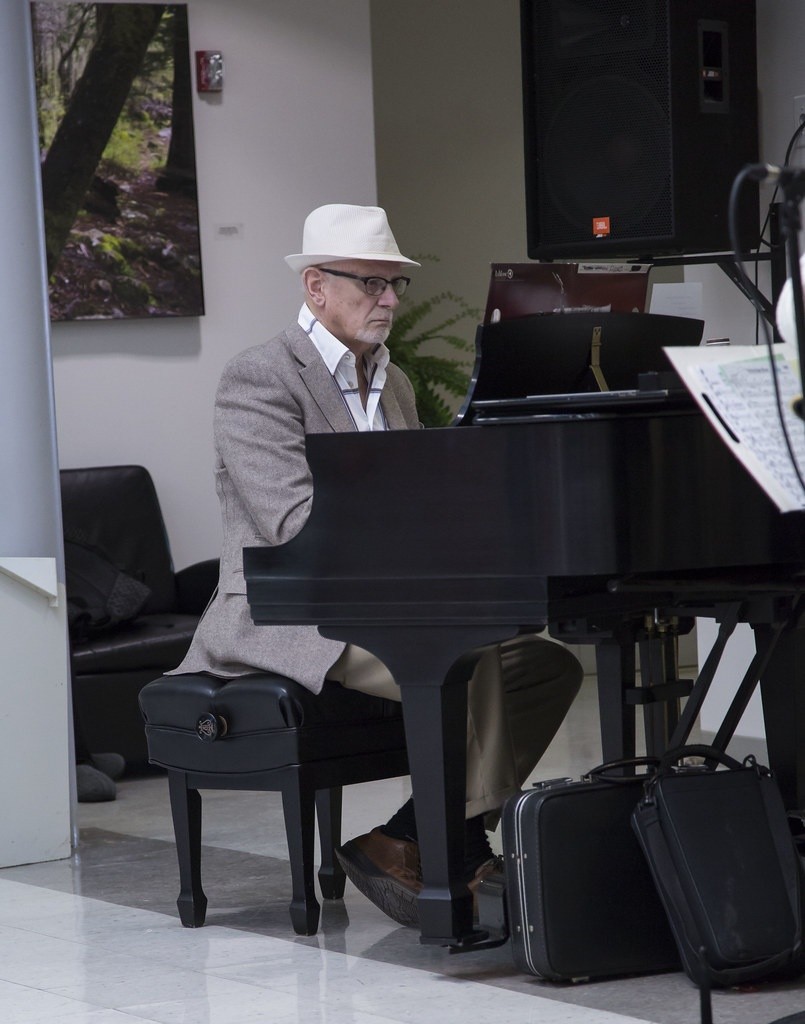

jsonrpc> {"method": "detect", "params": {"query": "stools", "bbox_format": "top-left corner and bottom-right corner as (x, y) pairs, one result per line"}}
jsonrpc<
(135, 664), (407, 936)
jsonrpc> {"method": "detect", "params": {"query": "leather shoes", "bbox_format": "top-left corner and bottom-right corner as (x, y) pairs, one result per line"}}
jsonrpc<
(469, 859), (507, 922)
(335, 826), (427, 929)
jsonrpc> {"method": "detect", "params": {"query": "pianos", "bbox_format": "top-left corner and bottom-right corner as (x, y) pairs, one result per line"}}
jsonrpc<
(241, 306), (804, 949)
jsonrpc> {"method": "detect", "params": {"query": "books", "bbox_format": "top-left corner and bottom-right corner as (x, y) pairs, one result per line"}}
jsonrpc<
(482, 260), (653, 324)
(659, 250), (805, 514)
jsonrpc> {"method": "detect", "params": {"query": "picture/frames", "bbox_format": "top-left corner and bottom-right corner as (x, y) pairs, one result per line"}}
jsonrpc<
(28, 0), (206, 323)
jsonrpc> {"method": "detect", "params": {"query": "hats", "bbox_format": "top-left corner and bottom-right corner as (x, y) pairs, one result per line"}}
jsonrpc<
(285, 203), (423, 272)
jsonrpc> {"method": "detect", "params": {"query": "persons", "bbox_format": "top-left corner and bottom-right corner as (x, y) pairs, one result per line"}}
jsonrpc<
(162, 204), (585, 929)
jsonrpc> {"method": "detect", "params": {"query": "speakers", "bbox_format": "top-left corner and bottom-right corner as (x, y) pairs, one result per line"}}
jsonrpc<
(518, 0), (759, 263)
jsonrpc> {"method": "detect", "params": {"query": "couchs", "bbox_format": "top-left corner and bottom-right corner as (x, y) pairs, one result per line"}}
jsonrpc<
(59, 462), (221, 770)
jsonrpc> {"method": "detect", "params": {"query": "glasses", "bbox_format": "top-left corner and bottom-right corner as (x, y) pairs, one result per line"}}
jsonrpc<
(319, 268), (411, 297)
